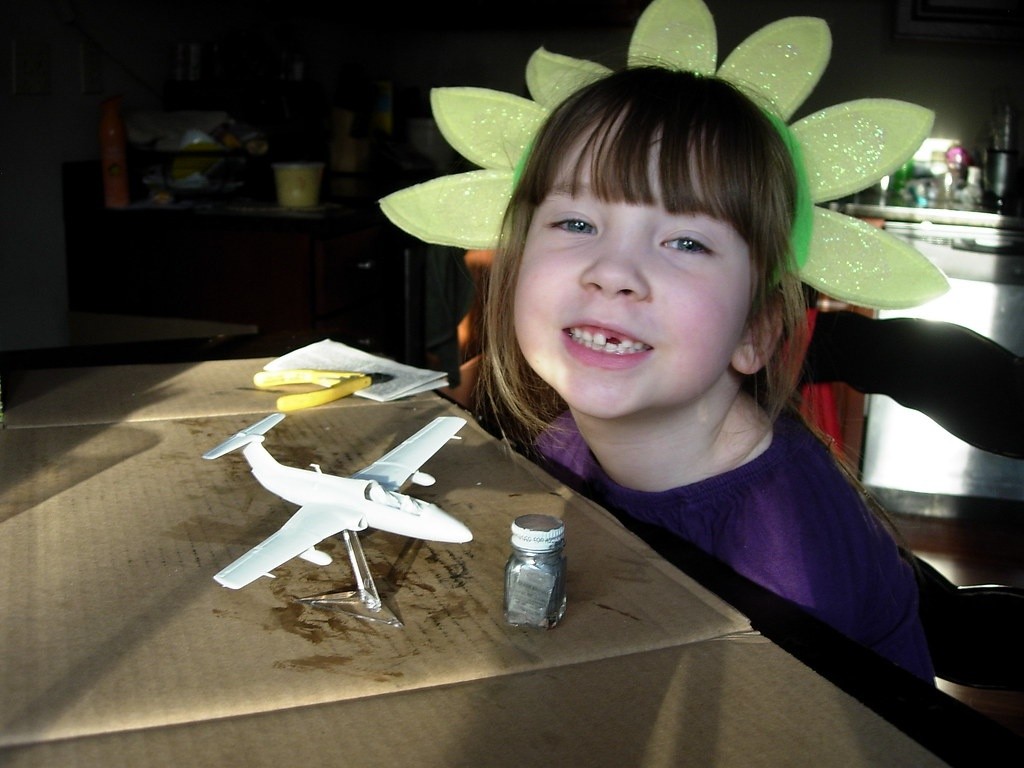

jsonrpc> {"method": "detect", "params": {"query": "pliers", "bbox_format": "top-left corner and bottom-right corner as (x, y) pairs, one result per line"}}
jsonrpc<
(252, 368), (398, 412)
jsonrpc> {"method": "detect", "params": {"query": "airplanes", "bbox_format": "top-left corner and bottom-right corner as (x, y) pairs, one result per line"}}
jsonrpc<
(202, 409), (477, 630)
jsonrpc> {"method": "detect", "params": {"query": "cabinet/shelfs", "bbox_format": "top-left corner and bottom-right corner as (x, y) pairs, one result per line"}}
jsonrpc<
(62, 149), (424, 363)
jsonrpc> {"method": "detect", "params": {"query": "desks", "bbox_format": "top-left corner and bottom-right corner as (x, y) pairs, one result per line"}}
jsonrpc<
(0, 357), (951, 768)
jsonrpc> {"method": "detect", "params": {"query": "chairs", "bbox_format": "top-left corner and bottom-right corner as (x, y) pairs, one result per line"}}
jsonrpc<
(773, 307), (1024, 768)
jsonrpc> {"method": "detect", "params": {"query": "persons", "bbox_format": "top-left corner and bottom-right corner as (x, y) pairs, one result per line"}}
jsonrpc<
(480, 66), (938, 688)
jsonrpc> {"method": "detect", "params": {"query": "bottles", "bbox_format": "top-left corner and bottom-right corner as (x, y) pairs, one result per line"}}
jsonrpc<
(506, 513), (567, 629)
(886, 161), (916, 206)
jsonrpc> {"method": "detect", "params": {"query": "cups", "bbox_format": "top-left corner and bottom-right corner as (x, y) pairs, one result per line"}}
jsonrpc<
(276, 164), (321, 208)
